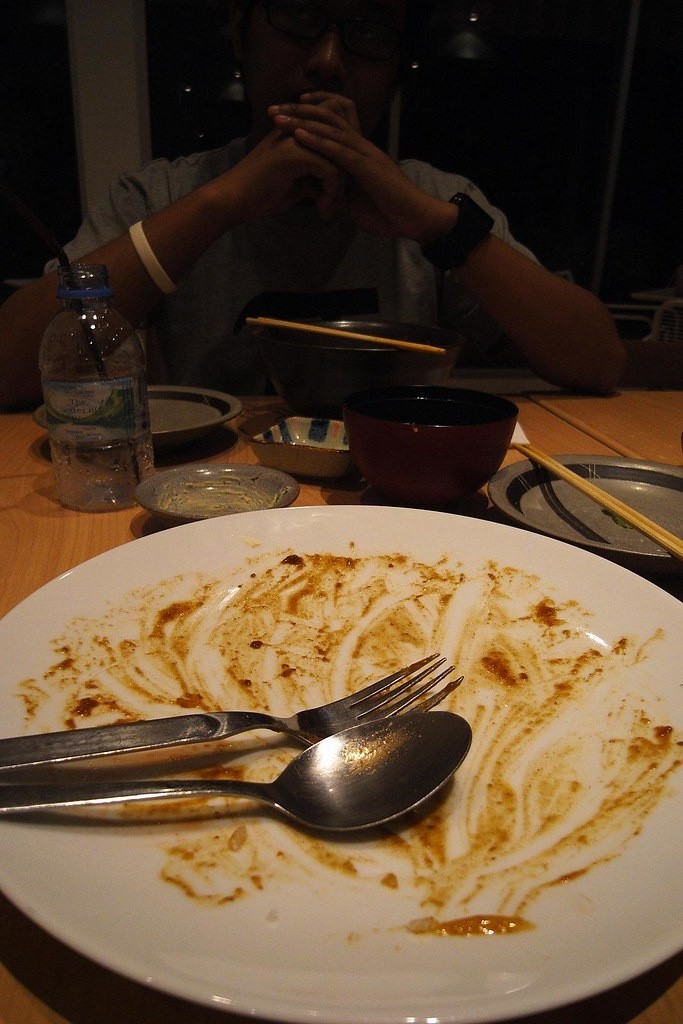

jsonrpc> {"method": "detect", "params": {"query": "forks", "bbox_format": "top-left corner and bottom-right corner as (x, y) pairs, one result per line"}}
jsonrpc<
(0, 653), (464, 769)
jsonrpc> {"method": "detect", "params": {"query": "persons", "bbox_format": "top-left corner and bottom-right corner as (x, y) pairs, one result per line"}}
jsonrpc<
(0, 0), (630, 410)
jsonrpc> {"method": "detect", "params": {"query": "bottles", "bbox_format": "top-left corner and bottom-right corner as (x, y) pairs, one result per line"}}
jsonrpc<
(39, 264), (154, 513)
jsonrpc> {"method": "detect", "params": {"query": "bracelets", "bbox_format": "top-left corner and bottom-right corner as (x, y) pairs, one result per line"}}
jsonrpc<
(129, 220), (178, 296)
(421, 191), (496, 273)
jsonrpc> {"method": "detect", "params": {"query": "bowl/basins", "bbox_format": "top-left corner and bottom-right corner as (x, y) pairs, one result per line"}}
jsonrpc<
(250, 417), (350, 477)
(258, 321), (452, 420)
(342, 385), (519, 504)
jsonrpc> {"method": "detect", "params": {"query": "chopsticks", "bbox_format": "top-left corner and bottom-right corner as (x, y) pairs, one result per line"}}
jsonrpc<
(245, 316), (447, 355)
(512, 443), (683, 561)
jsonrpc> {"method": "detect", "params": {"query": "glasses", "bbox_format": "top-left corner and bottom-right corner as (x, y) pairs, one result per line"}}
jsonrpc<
(256, 0), (405, 65)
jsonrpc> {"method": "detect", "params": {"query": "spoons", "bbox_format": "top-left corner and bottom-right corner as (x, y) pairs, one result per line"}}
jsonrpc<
(0, 711), (473, 832)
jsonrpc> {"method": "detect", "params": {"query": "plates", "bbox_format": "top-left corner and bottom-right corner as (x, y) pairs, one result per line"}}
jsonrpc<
(136, 463), (301, 528)
(0, 504), (683, 1024)
(34, 386), (244, 450)
(488, 455), (683, 574)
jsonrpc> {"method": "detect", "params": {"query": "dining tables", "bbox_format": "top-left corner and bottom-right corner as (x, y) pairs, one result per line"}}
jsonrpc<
(0, 339), (683, 1024)
